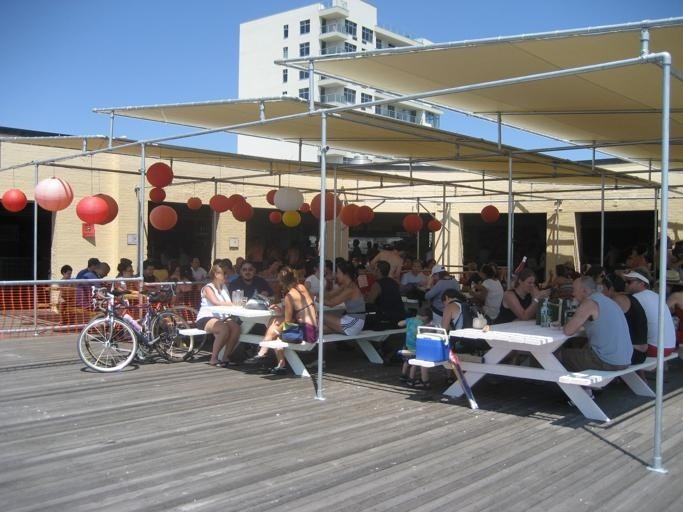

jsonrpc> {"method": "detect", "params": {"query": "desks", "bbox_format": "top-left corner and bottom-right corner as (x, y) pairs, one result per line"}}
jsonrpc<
(442, 316), (612, 420)
(199, 304), (379, 377)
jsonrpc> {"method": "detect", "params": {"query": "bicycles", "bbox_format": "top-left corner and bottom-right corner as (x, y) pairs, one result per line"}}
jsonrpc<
(78, 291), (193, 369)
(97, 292), (208, 361)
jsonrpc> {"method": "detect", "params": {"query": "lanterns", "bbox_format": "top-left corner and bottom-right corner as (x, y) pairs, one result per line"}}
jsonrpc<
(145, 162), (174, 188)
(2, 188), (27, 211)
(76, 193), (119, 225)
(186, 196), (203, 210)
(481, 205), (500, 222)
(148, 204), (179, 230)
(209, 186), (375, 229)
(149, 188), (166, 202)
(34, 176), (74, 213)
(402, 214), (422, 232)
(427, 219), (441, 232)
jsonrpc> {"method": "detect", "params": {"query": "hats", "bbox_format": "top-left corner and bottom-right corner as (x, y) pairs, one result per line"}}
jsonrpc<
(656, 269), (683, 286)
(430, 265), (446, 278)
(154, 269), (169, 282)
(624, 271), (649, 284)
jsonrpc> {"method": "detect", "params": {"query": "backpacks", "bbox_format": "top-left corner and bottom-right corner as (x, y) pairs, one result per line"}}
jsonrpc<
(449, 300), (491, 355)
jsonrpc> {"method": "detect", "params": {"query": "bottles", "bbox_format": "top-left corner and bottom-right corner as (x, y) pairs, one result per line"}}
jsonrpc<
(540, 302), (551, 328)
(515, 255), (528, 273)
(564, 298), (579, 323)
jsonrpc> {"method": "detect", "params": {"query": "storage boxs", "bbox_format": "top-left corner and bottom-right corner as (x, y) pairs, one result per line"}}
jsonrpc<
(415, 326), (449, 362)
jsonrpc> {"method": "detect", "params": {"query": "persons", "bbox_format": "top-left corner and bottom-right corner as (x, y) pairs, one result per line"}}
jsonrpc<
(58, 238), (682, 407)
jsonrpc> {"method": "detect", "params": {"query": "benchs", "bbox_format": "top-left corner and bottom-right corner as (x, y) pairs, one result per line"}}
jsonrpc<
(409, 355), (455, 370)
(179, 326), (206, 336)
(559, 352), (678, 420)
(258, 326), (405, 377)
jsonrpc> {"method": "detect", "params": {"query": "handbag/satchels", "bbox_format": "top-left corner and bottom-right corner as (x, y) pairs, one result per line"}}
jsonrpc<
(282, 322), (304, 343)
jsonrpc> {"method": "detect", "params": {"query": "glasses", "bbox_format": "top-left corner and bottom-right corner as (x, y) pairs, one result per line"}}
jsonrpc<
(242, 267), (255, 272)
(625, 279), (638, 285)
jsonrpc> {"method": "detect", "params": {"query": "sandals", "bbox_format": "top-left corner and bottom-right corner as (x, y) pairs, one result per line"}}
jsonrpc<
(399, 374), (454, 388)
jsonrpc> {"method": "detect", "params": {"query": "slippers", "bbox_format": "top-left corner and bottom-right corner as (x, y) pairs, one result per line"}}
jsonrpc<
(210, 356), (326, 374)
